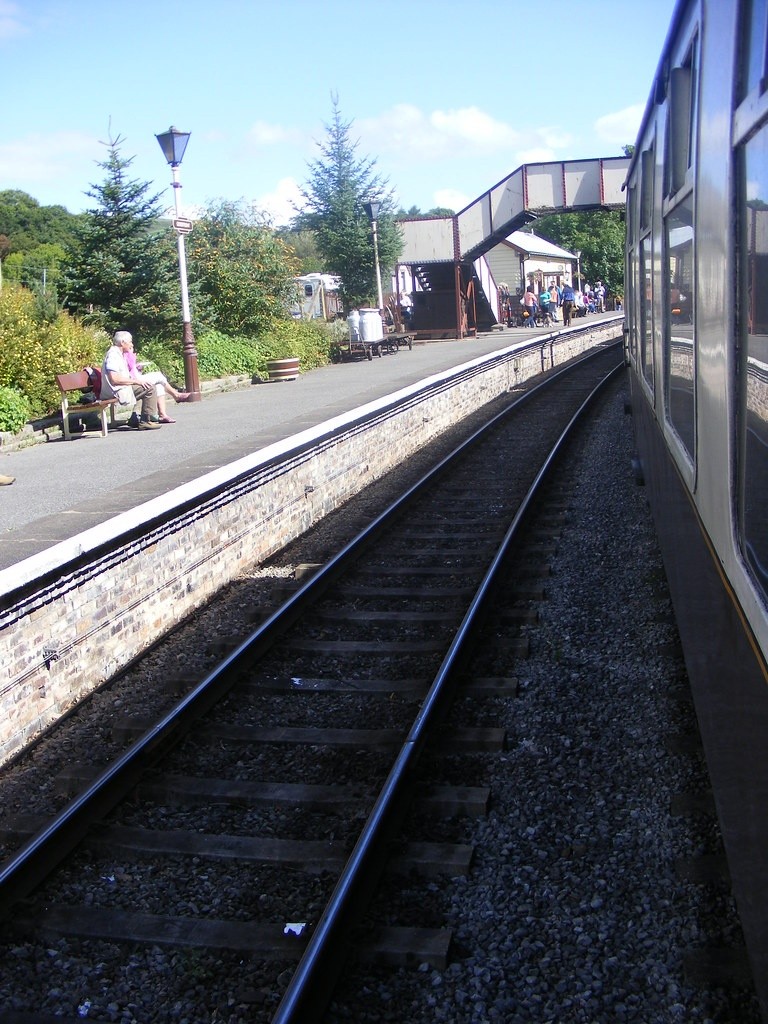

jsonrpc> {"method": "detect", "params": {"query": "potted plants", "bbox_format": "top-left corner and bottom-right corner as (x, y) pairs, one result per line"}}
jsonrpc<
(265, 341), (300, 380)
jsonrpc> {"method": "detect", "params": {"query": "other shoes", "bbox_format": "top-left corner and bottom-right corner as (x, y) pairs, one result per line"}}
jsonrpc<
(158, 416), (176, 423)
(139, 421), (161, 430)
(176, 393), (190, 403)
(555, 320), (558, 322)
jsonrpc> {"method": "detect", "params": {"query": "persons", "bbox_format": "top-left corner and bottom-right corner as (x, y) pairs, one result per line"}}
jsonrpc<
(99, 330), (162, 431)
(670, 283), (680, 326)
(123, 343), (190, 423)
(645, 278), (651, 313)
(616, 296), (622, 311)
(500, 279), (605, 329)
(394, 289), (412, 314)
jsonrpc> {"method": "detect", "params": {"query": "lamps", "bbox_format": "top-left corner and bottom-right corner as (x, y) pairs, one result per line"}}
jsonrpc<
(565, 270), (570, 281)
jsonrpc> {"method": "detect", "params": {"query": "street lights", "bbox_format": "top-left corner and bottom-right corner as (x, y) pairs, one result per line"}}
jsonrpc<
(153, 124), (205, 406)
(361, 196), (388, 332)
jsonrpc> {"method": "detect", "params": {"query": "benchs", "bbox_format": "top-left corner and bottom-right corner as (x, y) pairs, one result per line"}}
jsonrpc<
(55, 366), (141, 440)
(588, 301), (602, 313)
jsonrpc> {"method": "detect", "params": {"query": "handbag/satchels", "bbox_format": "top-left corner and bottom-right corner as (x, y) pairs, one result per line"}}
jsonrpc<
(79, 393), (96, 405)
(520, 297), (526, 306)
